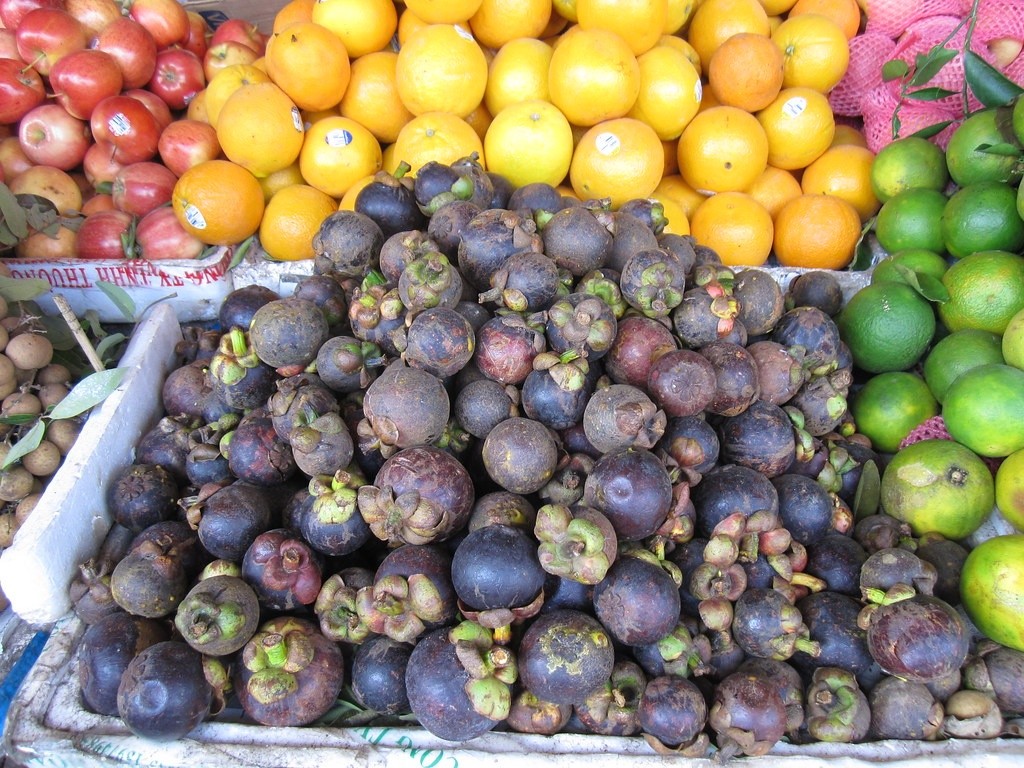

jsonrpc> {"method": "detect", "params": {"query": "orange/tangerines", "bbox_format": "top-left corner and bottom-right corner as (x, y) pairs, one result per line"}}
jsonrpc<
(839, 88), (1024, 651)
(168, 0), (883, 274)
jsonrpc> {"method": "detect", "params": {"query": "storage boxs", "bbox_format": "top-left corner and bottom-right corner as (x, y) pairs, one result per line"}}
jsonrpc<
(3, 255), (1023, 768)
(0, 244), (236, 324)
(0, 297), (183, 630)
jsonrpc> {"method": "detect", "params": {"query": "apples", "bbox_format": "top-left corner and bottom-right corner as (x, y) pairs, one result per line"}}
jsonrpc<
(0, 0), (272, 261)
